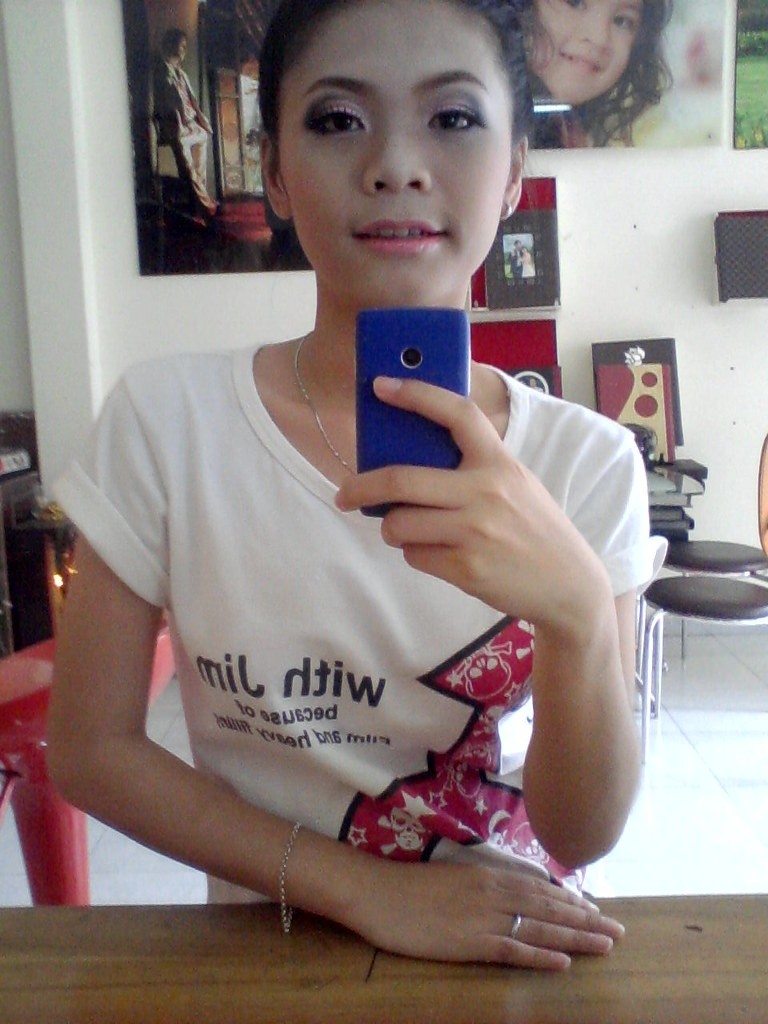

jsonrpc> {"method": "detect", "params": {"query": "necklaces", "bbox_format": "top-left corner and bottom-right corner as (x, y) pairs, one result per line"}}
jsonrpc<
(295, 331), (357, 475)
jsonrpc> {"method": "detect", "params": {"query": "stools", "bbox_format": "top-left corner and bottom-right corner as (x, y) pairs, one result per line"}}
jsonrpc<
(0, 617), (176, 904)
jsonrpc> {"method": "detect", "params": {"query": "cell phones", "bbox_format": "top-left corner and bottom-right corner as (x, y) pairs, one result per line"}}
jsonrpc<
(357, 306), (472, 519)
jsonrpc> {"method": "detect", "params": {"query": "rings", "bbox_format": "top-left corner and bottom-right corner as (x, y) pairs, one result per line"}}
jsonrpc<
(510, 914), (522, 939)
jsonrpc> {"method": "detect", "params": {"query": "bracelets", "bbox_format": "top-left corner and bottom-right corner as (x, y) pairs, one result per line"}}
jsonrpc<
(280, 821), (304, 933)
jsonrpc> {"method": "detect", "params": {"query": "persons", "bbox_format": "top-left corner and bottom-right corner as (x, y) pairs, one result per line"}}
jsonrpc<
(520, 377), (543, 392)
(153, 29), (219, 216)
(509, 241), (535, 278)
(523, 0), (672, 148)
(46, 0), (670, 969)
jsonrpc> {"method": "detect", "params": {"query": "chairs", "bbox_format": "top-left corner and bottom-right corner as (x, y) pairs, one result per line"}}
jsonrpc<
(641, 433), (768, 766)
(659, 539), (768, 675)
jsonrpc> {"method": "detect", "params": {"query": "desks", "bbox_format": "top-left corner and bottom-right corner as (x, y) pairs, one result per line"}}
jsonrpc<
(0, 893), (768, 1024)
